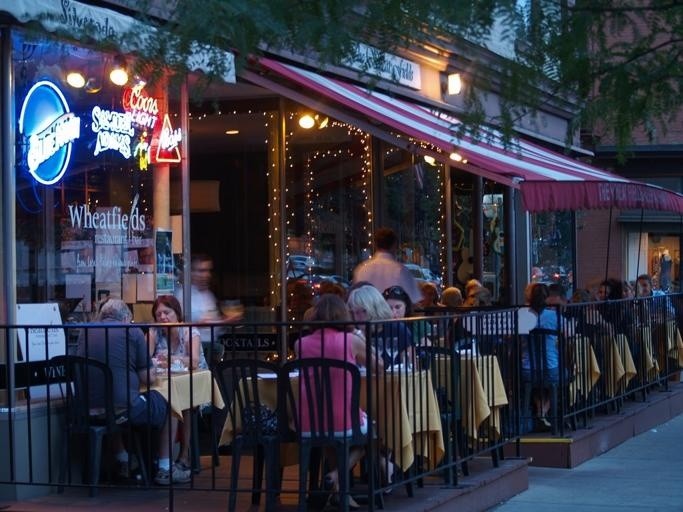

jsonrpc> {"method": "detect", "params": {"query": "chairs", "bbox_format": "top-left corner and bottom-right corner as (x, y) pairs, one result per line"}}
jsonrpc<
(41, 286), (682, 512)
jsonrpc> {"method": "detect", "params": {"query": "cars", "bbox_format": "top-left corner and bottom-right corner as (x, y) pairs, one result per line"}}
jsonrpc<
(288, 255), (442, 296)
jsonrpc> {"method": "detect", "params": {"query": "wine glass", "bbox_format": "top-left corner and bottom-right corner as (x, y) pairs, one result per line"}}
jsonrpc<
(385, 336), (399, 363)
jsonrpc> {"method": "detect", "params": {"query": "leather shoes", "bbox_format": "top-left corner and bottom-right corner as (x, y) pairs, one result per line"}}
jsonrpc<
(321, 471), (361, 508)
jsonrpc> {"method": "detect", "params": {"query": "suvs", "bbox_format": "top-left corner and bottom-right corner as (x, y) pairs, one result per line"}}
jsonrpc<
(540, 265), (568, 285)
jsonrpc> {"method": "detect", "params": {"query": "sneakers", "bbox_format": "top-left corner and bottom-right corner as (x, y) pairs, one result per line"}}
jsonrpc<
(113, 450), (140, 479)
(155, 465), (191, 485)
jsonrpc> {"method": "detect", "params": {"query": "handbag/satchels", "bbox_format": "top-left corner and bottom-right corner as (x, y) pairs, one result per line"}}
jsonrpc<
(242, 401), (277, 432)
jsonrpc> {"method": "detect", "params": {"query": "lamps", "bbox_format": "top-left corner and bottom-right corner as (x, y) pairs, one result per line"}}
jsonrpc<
(439, 68), (463, 96)
(293, 104), (328, 130)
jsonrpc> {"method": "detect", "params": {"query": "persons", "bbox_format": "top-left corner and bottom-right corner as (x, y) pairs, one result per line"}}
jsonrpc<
(637, 275), (675, 320)
(74, 295), (209, 485)
(548, 284), (568, 304)
(383, 286), (437, 346)
(574, 279), (650, 334)
(441, 286), (463, 305)
(449, 279), (489, 349)
(417, 283), (437, 309)
(189, 254), (243, 348)
(347, 285), (416, 369)
(354, 233), (424, 310)
(511, 283), (573, 425)
(304, 281), (346, 320)
(297, 295), (383, 506)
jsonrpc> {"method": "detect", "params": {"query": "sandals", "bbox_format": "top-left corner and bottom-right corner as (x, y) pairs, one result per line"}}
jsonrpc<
(175, 459), (192, 470)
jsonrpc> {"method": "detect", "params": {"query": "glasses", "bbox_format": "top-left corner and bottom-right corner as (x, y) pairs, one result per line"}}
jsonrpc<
(382, 286), (403, 300)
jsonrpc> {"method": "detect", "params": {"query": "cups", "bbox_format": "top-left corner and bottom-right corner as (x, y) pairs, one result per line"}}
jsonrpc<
(368, 337), (383, 357)
